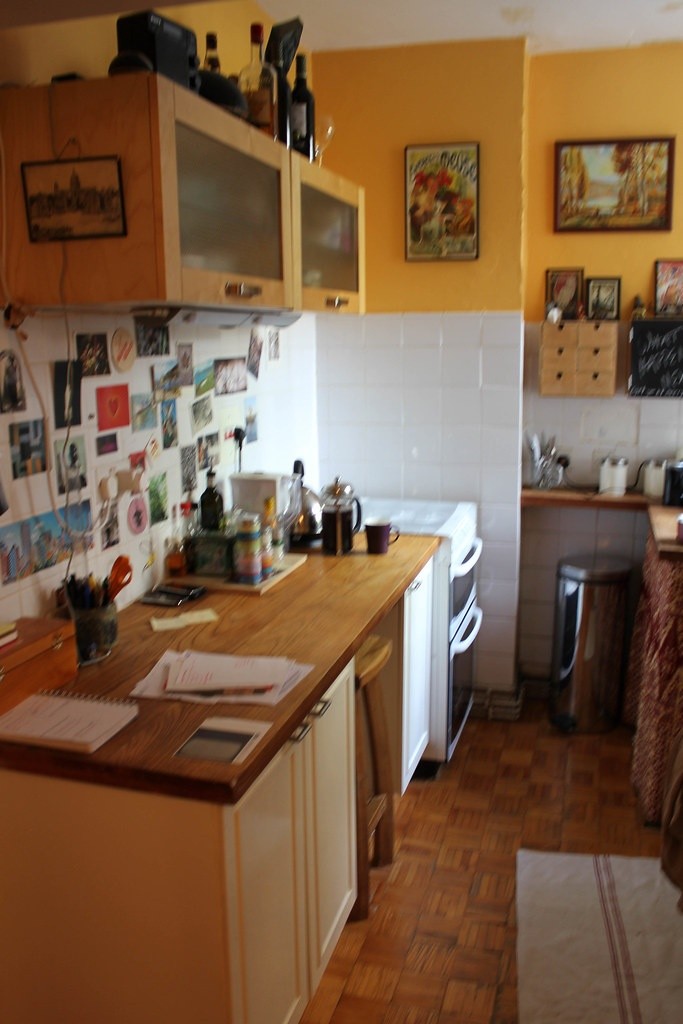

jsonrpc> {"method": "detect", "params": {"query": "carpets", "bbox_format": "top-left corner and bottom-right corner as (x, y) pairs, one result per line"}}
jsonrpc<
(515, 849), (682, 1024)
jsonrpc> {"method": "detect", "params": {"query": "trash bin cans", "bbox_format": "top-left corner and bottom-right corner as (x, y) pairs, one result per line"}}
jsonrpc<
(551, 554), (633, 729)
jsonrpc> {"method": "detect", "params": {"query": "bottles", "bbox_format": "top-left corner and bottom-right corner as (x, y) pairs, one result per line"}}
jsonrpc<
(237, 22), (279, 142)
(270, 46), (293, 148)
(201, 473), (224, 530)
(233, 497), (285, 584)
(202, 31), (220, 75)
(291, 54), (315, 165)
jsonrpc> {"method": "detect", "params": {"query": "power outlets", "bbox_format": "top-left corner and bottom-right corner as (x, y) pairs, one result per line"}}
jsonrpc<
(99, 458), (132, 506)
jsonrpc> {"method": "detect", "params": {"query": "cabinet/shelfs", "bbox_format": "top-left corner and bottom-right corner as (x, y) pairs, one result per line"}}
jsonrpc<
(0, 72), (367, 316)
(537, 320), (617, 398)
(0, 532), (441, 1024)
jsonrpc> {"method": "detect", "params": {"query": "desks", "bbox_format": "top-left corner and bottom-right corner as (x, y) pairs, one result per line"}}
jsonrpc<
(520, 487), (683, 831)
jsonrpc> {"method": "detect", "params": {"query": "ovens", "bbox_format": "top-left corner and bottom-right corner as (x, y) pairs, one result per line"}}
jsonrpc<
(351, 535), (483, 777)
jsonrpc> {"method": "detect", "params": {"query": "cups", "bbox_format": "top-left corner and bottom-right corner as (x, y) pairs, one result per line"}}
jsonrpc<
(531, 454), (564, 490)
(643, 460), (665, 498)
(68, 601), (119, 668)
(663, 462), (683, 505)
(599, 456), (628, 496)
(363, 519), (400, 554)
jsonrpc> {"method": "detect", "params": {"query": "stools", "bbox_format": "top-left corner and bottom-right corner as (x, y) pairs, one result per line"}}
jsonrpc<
(355, 635), (393, 920)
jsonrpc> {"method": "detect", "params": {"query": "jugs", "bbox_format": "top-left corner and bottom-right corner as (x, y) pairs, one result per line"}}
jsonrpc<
(229, 469), (302, 555)
(319, 475), (362, 556)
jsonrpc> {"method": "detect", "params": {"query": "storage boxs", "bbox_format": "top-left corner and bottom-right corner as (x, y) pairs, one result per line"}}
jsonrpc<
(0, 615), (80, 717)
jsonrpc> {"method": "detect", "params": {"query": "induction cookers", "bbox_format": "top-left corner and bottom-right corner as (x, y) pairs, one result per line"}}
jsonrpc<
(312, 490), (477, 555)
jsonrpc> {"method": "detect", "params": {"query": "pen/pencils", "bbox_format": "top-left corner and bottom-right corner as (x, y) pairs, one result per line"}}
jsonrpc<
(62, 572), (109, 610)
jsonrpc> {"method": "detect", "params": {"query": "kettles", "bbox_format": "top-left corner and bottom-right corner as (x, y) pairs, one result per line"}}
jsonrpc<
(285, 460), (322, 545)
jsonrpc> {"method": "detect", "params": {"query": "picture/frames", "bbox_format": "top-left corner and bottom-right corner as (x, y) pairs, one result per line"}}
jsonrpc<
(404, 141), (479, 262)
(544, 268), (586, 320)
(587, 278), (620, 320)
(555, 134), (675, 234)
(654, 260), (683, 318)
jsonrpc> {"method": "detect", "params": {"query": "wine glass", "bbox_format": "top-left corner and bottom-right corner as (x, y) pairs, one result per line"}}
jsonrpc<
(314, 113), (336, 167)
